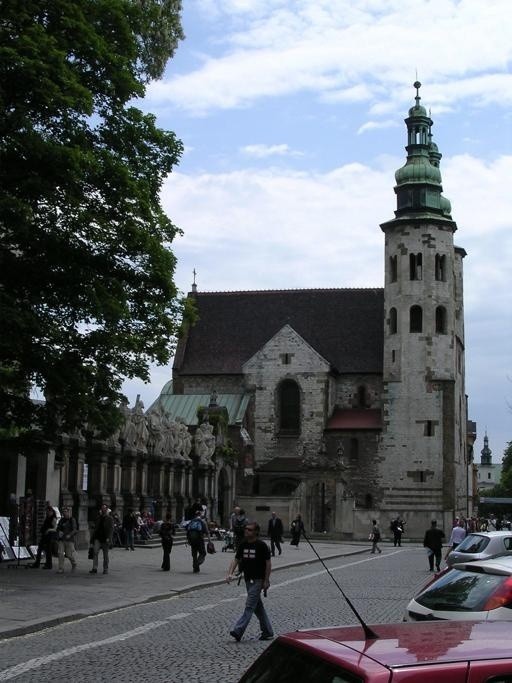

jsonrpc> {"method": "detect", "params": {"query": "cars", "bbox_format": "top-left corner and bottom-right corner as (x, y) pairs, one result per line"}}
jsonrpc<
(232, 620), (512, 682)
(441, 528), (512, 566)
(400, 555), (511, 621)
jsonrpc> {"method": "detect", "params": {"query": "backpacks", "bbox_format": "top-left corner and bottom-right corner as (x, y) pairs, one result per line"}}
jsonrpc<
(187, 522), (203, 545)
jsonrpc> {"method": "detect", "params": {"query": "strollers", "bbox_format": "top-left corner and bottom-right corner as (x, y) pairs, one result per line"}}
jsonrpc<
(221, 530), (237, 553)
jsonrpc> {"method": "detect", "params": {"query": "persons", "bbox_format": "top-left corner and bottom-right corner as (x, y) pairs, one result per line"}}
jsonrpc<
(29, 506), (56, 569)
(391, 518), (404, 546)
(449, 514), (512, 551)
(109, 400), (216, 466)
(268, 512), (282, 556)
(371, 520), (381, 553)
(226, 522), (273, 641)
(56, 507), (77, 574)
(290, 514), (305, 547)
(424, 520), (445, 571)
(89, 498), (248, 574)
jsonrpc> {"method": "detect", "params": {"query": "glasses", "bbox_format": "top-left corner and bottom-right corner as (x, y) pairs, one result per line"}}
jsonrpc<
(245, 526), (254, 532)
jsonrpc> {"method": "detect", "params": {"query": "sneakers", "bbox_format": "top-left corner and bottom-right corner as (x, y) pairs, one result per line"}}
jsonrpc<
(258, 633), (274, 640)
(230, 630), (241, 641)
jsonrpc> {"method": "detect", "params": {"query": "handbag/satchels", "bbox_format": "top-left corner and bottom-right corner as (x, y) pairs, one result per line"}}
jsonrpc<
(88, 548), (94, 559)
